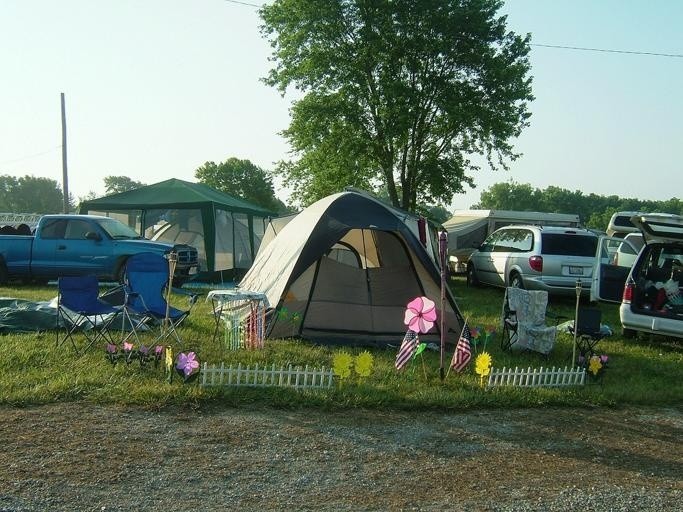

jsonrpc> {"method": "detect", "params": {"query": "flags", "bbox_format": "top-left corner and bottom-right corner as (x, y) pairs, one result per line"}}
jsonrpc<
(394, 324), (426, 370)
(447, 320), (473, 375)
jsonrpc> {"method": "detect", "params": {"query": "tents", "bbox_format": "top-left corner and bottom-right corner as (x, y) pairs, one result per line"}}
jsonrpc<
(250, 186), (442, 268)
(212, 189), (472, 350)
(77, 177), (278, 282)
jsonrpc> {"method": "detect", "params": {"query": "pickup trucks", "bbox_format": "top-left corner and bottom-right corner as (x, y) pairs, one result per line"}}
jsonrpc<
(0, 215), (198, 288)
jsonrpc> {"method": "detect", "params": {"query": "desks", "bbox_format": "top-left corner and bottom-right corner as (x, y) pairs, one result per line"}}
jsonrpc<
(205, 290), (270, 352)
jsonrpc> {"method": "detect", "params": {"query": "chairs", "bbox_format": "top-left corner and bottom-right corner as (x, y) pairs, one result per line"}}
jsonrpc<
(501, 287), (567, 363)
(55, 276), (124, 354)
(122, 253), (205, 353)
(569, 310), (613, 363)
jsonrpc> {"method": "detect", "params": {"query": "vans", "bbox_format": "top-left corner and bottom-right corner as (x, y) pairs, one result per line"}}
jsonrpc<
(467, 211), (682, 338)
